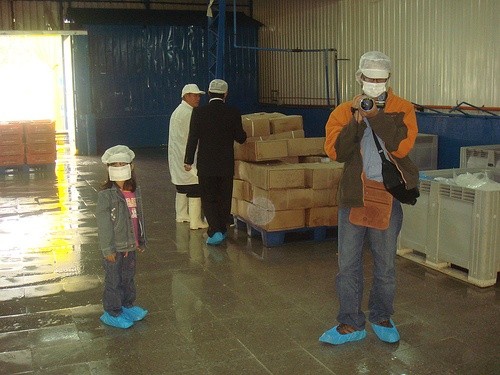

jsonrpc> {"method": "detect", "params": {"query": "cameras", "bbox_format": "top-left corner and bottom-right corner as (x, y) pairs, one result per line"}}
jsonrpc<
(360, 97), (385, 111)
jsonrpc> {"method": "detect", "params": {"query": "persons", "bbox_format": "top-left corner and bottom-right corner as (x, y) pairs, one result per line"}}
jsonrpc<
(319, 51), (419, 346)
(184, 78), (248, 246)
(96, 145), (149, 329)
(168, 83), (210, 230)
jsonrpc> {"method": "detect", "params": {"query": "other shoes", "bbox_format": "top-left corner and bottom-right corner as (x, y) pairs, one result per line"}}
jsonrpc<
(121, 305), (147, 321)
(206, 231), (224, 246)
(318, 321), (368, 346)
(370, 317), (402, 344)
(99, 311), (135, 329)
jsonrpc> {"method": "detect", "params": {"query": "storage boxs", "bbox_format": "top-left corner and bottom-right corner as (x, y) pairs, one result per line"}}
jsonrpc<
(231, 113), (346, 232)
(0, 120), (57, 165)
(406, 133), (500, 286)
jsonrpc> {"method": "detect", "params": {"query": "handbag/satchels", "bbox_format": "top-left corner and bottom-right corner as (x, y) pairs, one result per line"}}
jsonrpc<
(381, 159), (421, 206)
(348, 177), (394, 231)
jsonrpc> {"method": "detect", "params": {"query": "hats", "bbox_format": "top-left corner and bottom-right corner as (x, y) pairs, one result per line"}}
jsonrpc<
(181, 83), (206, 99)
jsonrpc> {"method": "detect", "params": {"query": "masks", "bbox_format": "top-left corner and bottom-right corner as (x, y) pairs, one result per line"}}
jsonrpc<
(361, 81), (386, 98)
(107, 164), (132, 182)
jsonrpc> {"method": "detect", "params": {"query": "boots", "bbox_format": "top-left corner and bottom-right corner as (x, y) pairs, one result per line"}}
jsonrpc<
(188, 197), (210, 230)
(174, 192), (190, 223)
